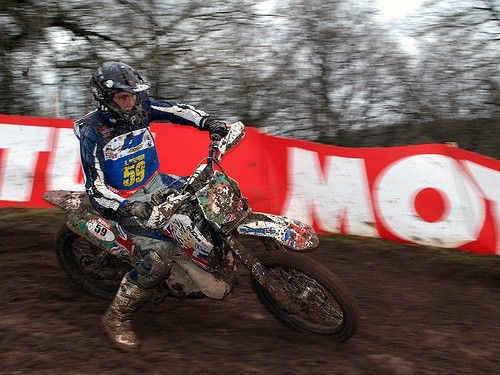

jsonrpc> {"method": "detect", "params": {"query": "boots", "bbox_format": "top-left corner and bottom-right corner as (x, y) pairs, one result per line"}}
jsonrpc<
(100, 269), (156, 354)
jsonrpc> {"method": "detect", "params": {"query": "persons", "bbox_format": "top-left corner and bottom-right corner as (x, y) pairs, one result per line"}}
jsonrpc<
(74, 61), (227, 349)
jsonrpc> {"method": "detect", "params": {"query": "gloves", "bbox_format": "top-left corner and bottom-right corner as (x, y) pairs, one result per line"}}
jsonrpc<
(116, 199), (152, 221)
(204, 116), (227, 135)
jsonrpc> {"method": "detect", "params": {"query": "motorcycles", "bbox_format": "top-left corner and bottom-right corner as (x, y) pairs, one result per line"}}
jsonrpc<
(44, 122), (359, 345)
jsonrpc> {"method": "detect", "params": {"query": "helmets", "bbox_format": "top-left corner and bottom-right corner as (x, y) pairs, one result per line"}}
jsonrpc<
(89, 61), (153, 128)
